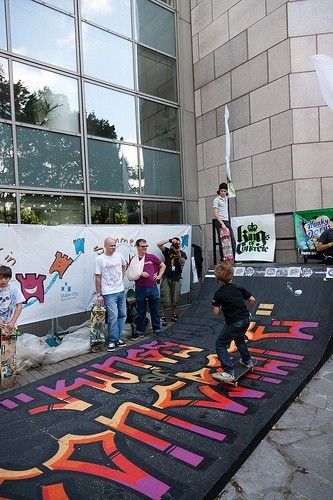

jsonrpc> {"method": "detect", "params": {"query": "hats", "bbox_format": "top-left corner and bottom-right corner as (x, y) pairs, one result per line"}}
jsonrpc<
(219, 183), (228, 190)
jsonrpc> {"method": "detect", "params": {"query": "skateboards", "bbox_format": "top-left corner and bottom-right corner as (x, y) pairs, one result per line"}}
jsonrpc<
(90, 302), (106, 352)
(39, 334), (64, 348)
(218, 358), (258, 387)
(219, 228), (234, 266)
(127, 288), (137, 324)
(0, 323), (21, 389)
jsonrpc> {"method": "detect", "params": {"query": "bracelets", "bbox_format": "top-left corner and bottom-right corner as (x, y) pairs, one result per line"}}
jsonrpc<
(97, 294), (102, 297)
(169, 239), (173, 243)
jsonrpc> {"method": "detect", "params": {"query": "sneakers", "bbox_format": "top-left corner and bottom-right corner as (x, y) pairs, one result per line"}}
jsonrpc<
(212, 372), (236, 381)
(131, 334), (145, 341)
(107, 343), (116, 352)
(116, 339), (126, 347)
(239, 358), (254, 368)
(171, 314), (178, 321)
(161, 316), (167, 326)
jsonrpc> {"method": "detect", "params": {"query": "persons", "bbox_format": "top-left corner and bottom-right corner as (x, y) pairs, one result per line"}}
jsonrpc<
(157, 236), (188, 326)
(212, 183), (242, 265)
(128, 239), (167, 340)
(211, 264), (256, 382)
(0, 265), (25, 330)
(94, 237), (127, 351)
(317, 219), (333, 266)
